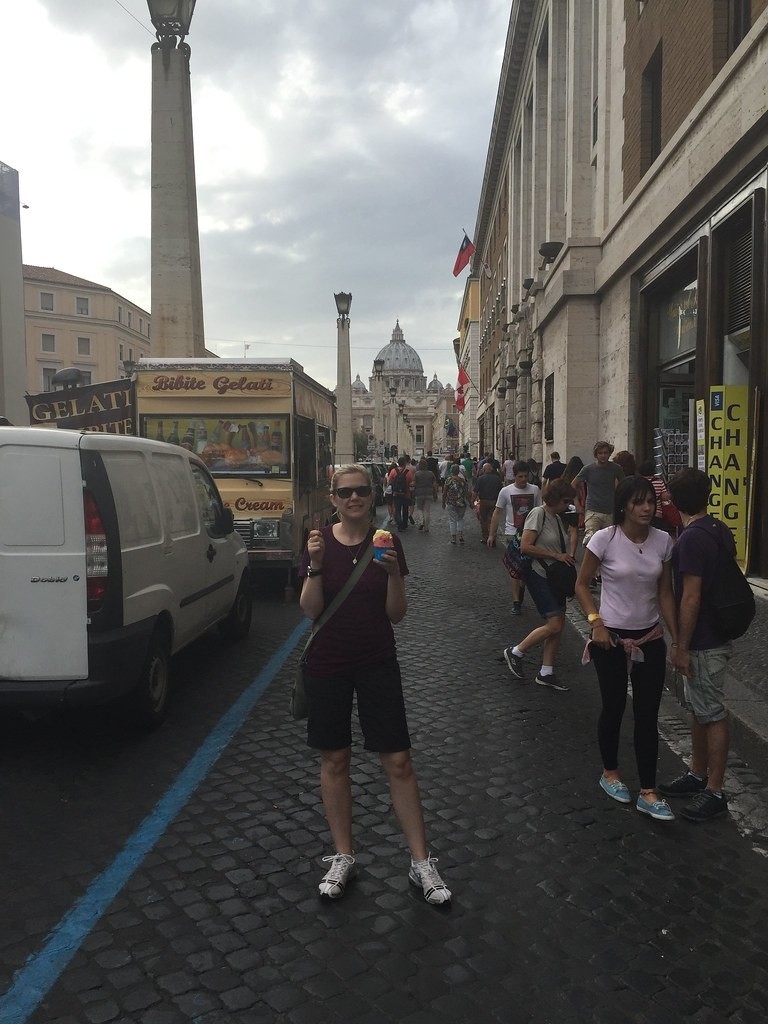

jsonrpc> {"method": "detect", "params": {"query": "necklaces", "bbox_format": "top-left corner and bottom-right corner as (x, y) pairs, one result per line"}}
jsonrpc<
(347, 543), (362, 564)
(627, 536), (646, 554)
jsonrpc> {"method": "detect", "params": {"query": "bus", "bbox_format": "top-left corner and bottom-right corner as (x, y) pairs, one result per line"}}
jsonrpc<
(136, 357), (337, 597)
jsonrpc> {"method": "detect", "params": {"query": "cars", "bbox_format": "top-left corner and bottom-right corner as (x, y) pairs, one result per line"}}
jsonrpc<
(358, 460), (399, 507)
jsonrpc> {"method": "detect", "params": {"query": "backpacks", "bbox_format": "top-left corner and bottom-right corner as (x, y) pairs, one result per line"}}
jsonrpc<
(392, 467), (409, 494)
(682, 521), (755, 642)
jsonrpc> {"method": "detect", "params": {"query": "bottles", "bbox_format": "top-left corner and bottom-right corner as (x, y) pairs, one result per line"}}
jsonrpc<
(210, 421), (223, 441)
(262, 426), (270, 444)
(156, 421), (165, 442)
(166, 421), (180, 446)
(197, 420), (207, 453)
(180, 420), (196, 451)
(248, 422), (261, 448)
(242, 425), (251, 450)
(271, 420), (281, 451)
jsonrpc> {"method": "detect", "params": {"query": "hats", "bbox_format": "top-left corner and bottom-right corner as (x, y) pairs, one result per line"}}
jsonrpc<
(450, 464), (460, 473)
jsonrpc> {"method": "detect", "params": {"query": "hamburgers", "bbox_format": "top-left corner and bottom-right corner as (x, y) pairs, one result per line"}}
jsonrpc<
(259, 450), (284, 468)
(224, 449), (249, 469)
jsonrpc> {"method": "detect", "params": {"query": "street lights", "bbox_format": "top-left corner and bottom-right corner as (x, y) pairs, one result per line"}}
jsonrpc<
(373, 358), (386, 454)
(387, 386), (398, 448)
(334, 292), (356, 466)
(397, 403), (415, 459)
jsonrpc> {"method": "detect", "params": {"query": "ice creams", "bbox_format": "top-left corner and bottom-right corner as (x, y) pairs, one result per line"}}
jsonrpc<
(372, 529), (394, 548)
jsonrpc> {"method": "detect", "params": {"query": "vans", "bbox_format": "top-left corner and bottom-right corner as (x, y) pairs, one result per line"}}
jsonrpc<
(0, 427), (253, 731)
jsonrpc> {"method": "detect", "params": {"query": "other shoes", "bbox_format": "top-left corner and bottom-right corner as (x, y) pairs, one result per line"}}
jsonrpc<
(481, 538), (487, 544)
(590, 579), (597, 588)
(511, 604), (520, 615)
(596, 577), (602, 584)
(450, 537), (456, 543)
(459, 537), (465, 544)
(384, 513), (432, 535)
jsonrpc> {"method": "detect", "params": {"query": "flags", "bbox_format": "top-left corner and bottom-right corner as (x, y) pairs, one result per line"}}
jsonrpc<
(455, 367), (469, 410)
(452, 235), (476, 277)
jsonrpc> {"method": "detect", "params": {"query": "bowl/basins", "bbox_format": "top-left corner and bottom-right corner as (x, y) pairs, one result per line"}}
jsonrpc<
(374, 547), (395, 561)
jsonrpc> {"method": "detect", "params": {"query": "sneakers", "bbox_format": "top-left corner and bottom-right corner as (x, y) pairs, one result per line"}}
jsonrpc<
(535, 671), (570, 691)
(599, 774), (631, 802)
(655, 767), (708, 796)
(679, 787), (728, 820)
(503, 647), (524, 679)
(636, 795), (674, 820)
(318, 853), (355, 900)
(409, 855), (452, 907)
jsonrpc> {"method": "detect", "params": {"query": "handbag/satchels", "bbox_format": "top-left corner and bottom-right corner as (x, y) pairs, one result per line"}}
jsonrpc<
(661, 500), (683, 527)
(503, 507), (546, 580)
(290, 655), (315, 719)
(546, 561), (576, 599)
(384, 485), (393, 501)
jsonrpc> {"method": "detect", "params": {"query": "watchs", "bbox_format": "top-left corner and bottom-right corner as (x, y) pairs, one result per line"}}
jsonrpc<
(307, 565), (322, 577)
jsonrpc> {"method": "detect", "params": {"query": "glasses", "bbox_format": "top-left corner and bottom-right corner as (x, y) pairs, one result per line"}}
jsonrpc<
(334, 485), (372, 499)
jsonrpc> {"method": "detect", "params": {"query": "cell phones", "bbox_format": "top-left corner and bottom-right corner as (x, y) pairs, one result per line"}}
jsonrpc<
(589, 627), (619, 647)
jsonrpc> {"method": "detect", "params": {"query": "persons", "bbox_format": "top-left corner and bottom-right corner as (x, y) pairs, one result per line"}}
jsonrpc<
(439, 452), (500, 493)
(383, 451), (440, 532)
(575, 475), (678, 820)
(298, 464), (452, 905)
(613, 451), (668, 530)
(527, 459), (543, 484)
(502, 452), (517, 485)
(542, 451), (567, 487)
(657, 467), (738, 821)
(441, 464), (473, 543)
(561, 456), (584, 558)
(471, 463), (503, 547)
(504, 479), (577, 690)
(572, 441), (625, 589)
(486, 461), (542, 616)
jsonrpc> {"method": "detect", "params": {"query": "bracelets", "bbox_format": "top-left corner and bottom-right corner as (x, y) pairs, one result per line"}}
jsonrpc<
(671, 643), (678, 648)
(587, 614), (600, 624)
